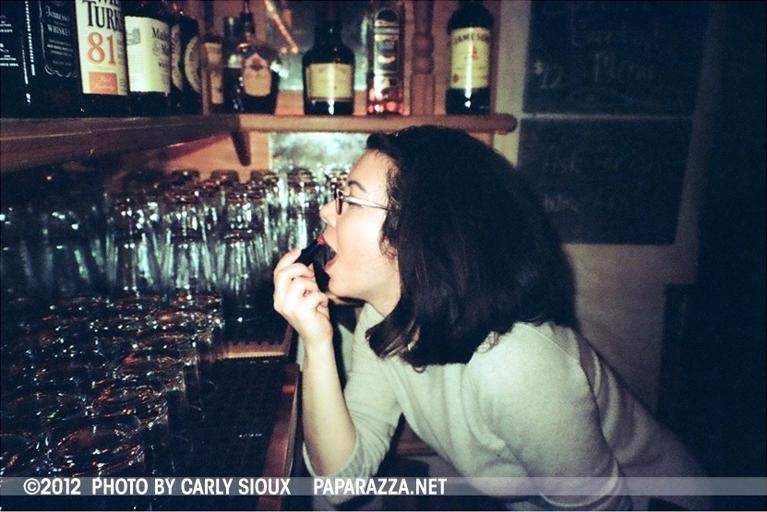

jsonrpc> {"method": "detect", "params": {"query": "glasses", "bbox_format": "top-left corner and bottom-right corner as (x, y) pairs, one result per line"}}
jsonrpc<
(333, 186), (400, 216)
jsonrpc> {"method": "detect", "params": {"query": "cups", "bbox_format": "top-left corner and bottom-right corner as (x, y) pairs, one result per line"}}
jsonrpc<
(0, 291), (223, 511)
(0, 168), (353, 319)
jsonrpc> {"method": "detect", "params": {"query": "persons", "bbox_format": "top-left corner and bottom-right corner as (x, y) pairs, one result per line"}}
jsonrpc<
(271, 124), (713, 511)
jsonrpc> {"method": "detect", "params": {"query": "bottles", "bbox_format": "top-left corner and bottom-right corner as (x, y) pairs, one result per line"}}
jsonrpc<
(366, 1), (405, 116)
(0, 1), (201, 117)
(304, 1), (356, 117)
(201, 0), (280, 113)
(446, 1), (493, 115)
